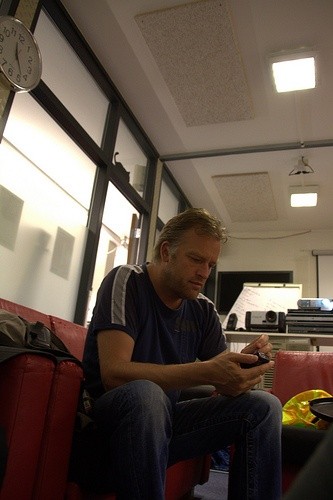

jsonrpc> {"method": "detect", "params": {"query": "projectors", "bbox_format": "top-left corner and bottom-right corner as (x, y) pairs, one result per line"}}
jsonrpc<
(245, 310), (286, 333)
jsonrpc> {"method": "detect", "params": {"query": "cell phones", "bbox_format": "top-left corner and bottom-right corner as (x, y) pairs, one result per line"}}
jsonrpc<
(240, 351), (270, 369)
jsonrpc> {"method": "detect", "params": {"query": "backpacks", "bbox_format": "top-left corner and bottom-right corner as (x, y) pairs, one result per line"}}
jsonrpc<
(0, 306), (95, 414)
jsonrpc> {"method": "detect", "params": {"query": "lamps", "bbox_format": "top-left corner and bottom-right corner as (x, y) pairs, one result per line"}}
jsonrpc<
(288, 155), (315, 176)
(288, 186), (318, 208)
(268, 50), (316, 94)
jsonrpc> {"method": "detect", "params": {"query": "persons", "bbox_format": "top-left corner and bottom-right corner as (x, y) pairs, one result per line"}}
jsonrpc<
(68, 207), (282, 500)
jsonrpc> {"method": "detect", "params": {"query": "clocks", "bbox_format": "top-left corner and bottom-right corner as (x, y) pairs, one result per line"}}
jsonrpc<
(0, 16), (43, 93)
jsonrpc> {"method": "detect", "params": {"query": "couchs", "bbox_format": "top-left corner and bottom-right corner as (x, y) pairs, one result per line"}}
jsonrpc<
(0, 297), (210, 500)
(223, 349), (333, 500)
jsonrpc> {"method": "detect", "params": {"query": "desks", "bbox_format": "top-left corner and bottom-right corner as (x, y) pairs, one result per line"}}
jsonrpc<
(223, 330), (333, 353)
